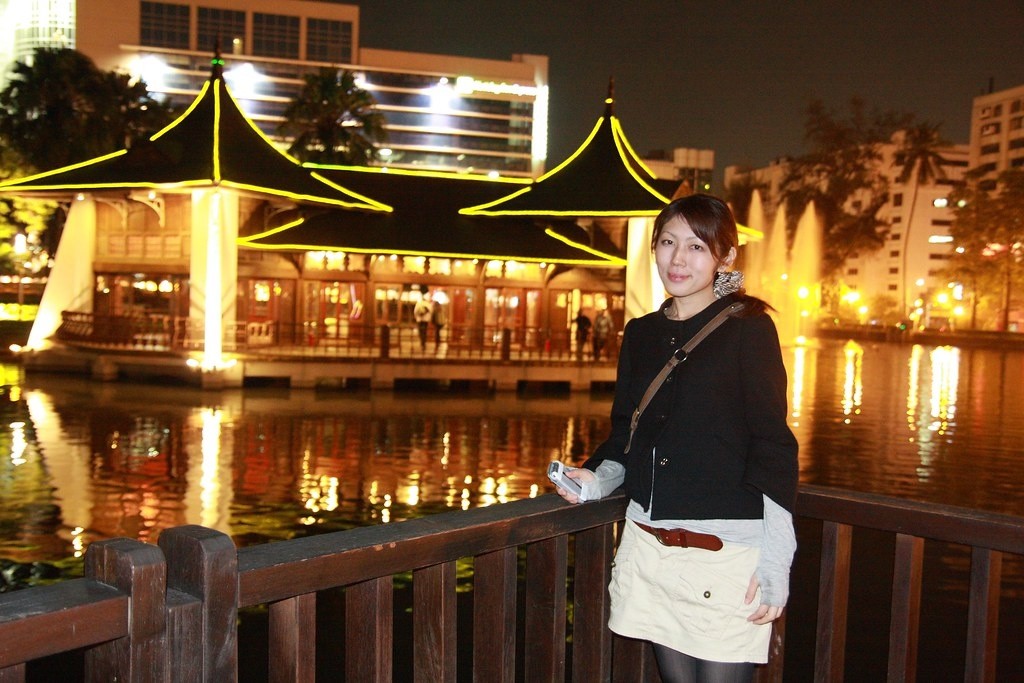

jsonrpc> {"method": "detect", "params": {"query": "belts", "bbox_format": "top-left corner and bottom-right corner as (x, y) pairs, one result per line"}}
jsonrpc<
(632, 521), (722, 552)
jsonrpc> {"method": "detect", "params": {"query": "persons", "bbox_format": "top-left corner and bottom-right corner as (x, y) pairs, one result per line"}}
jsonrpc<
(555, 194), (800, 683)
(414, 292), (445, 353)
(593, 309), (610, 362)
(571, 309), (591, 360)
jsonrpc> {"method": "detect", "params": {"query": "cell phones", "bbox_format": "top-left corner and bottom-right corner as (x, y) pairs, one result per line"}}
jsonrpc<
(548, 461), (587, 504)
